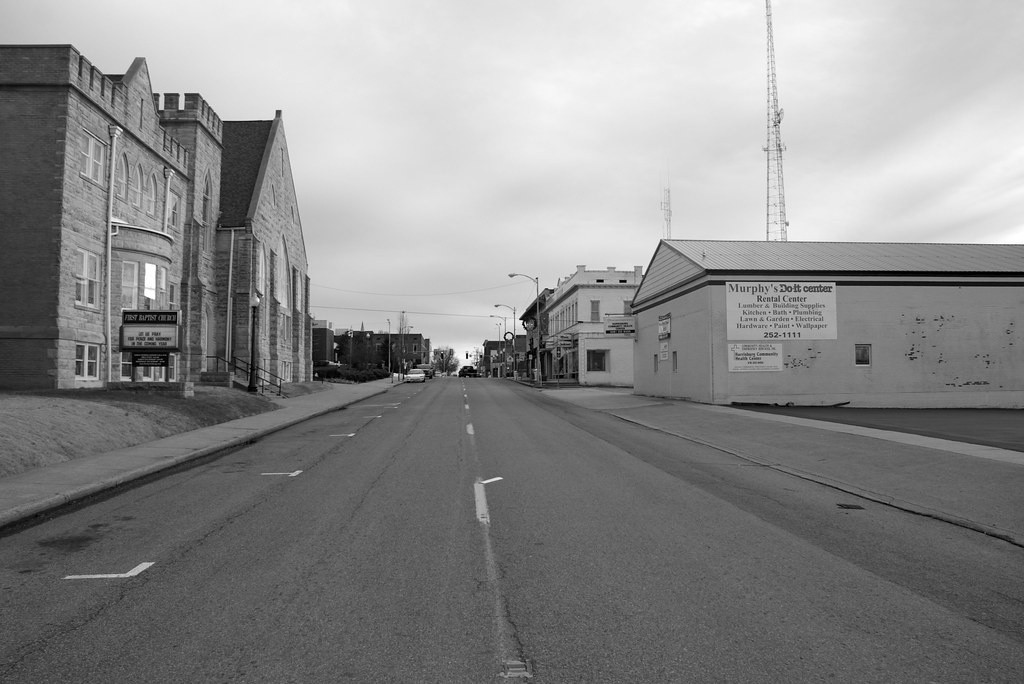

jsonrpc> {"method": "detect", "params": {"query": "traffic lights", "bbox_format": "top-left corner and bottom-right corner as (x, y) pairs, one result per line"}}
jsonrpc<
(479, 355), (482, 358)
(466, 353), (468, 359)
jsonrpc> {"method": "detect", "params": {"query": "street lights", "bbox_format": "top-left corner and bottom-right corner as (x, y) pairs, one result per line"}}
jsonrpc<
(490, 314), (507, 379)
(508, 273), (542, 388)
(247, 293), (260, 392)
(495, 304), (516, 383)
(402, 326), (414, 380)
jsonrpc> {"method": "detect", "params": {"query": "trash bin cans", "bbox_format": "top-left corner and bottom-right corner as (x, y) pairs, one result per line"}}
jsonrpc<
(531, 368), (538, 382)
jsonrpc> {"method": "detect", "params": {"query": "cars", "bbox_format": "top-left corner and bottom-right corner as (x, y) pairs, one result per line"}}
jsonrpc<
(406, 369), (426, 383)
(458, 366), (478, 378)
(417, 364), (434, 379)
